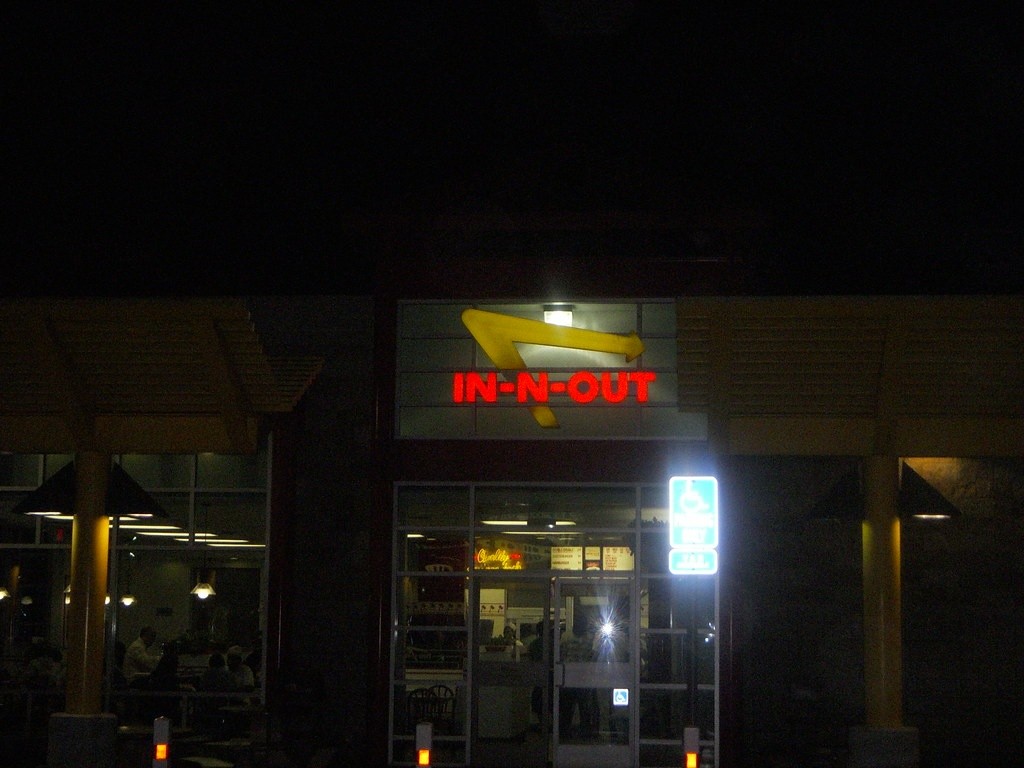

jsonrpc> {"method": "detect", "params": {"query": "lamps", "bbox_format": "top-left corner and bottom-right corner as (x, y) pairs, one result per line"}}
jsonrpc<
(189, 502), (217, 600)
(544, 306), (572, 327)
(119, 554), (137, 607)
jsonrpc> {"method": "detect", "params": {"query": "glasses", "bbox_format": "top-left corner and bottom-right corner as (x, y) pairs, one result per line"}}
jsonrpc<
(150, 633), (156, 637)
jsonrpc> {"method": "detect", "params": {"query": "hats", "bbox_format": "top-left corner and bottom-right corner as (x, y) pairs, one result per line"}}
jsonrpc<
(504, 623), (516, 632)
(141, 624), (158, 634)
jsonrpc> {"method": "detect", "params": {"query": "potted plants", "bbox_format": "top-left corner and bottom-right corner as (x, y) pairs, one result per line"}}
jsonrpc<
(484, 635), (507, 652)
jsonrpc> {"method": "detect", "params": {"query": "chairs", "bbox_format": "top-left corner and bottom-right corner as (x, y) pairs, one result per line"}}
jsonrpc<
(400, 684), (462, 761)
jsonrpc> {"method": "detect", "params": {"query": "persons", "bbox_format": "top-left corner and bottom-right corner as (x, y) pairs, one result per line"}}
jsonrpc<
(527, 596), (648, 737)
(503, 622), (528, 661)
(196, 645), (255, 692)
(123, 625), (163, 675)
